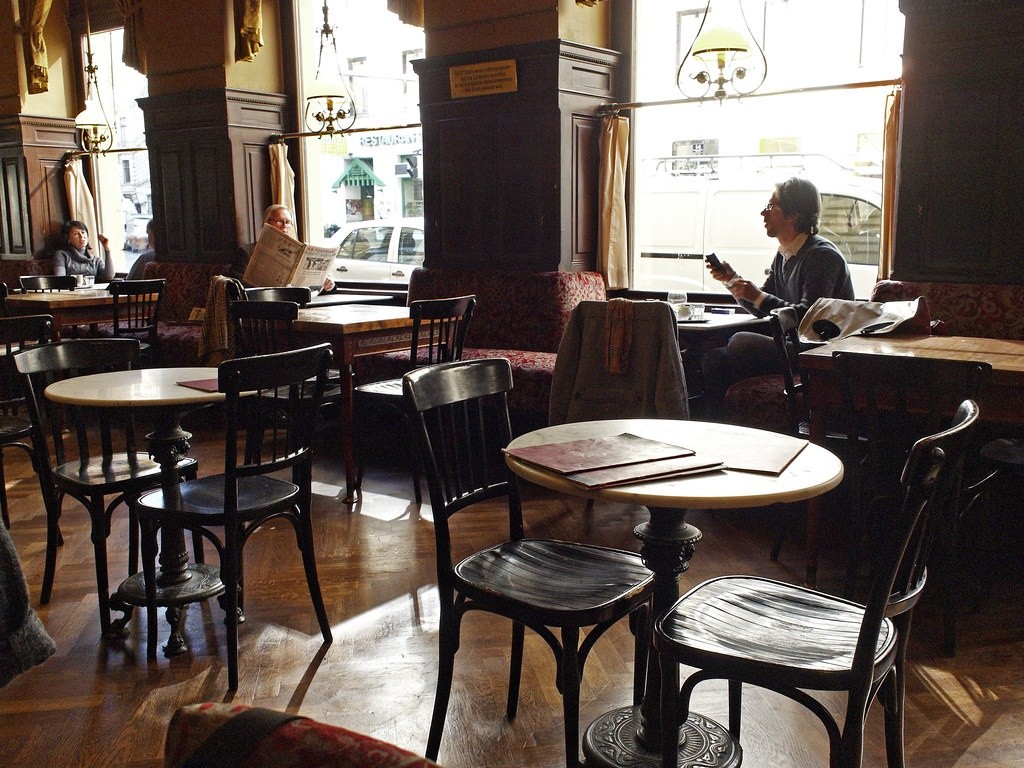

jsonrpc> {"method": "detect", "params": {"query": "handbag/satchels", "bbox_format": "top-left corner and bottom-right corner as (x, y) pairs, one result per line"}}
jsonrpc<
(796, 295), (949, 345)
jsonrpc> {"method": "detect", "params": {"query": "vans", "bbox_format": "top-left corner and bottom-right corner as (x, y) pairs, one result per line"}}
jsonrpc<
(625, 151), (882, 300)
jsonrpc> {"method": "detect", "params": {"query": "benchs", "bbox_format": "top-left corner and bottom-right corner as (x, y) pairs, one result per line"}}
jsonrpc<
(66, 261), (231, 366)
(354, 267), (606, 415)
(0, 259), (54, 289)
(722, 281), (1024, 435)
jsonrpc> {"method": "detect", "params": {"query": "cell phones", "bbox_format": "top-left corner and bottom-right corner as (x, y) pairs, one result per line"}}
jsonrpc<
(706, 253), (726, 275)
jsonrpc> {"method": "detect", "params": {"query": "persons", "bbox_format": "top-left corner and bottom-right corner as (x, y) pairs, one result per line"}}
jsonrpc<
(124, 218), (157, 280)
(702, 177), (854, 419)
(234, 202), (338, 295)
(52, 220), (115, 284)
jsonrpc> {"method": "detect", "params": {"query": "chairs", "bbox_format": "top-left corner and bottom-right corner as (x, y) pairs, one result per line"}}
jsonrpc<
(0, 276), (1024, 768)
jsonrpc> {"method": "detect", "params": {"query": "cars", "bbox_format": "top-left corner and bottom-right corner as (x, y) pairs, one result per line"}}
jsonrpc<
(321, 217), (424, 283)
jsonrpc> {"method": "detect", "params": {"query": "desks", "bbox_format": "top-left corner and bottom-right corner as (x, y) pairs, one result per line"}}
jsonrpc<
(246, 304), (463, 504)
(798, 332), (1023, 591)
(506, 418), (844, 768)
(678, 313), (773, 421)
(44, 366), (268, 654)
(4, 293), (159, 433)
(307, 294), (394, 307)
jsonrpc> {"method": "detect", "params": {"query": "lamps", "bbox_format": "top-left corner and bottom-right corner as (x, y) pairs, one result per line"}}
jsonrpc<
(75, 0), (113, 158)
(677, 0), (767, 105)
(304, 0), (357, 140)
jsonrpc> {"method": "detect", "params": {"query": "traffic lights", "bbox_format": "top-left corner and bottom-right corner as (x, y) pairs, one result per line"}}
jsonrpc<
(406, 155), (419, 179)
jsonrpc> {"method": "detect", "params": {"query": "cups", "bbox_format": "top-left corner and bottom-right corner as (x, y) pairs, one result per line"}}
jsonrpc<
(688, 303), (705, 321)
(72, 274), (83, 286)
(84, 275), (95, 285)
(668, 290), (687, 313)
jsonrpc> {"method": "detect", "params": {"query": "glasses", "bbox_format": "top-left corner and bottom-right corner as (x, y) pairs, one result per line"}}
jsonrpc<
(764, 202), (779, 212)
(268, 218), (294, 228)
